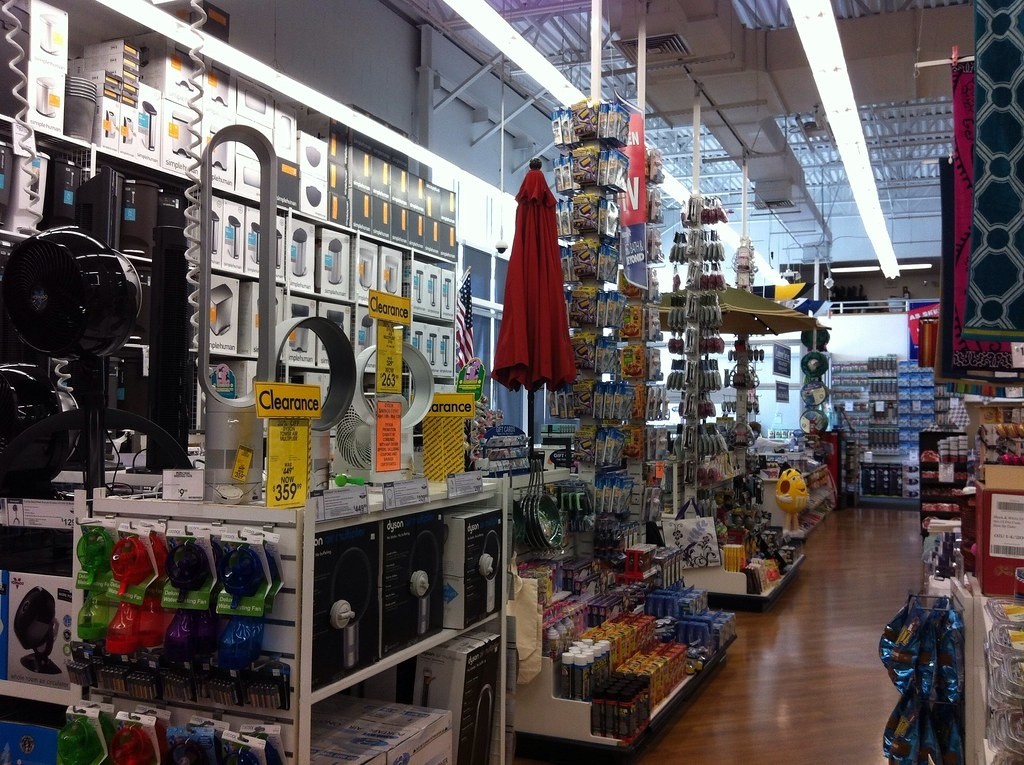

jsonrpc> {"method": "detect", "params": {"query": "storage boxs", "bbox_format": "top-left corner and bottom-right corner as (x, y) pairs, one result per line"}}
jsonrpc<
(0, 715), (63, 765)
(0, 3), (456, 422)
(0, 565), (73, 689)
(310, 501), (503, 765)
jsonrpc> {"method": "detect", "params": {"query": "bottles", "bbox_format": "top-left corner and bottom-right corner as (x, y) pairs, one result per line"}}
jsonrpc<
(542, 616), (650, 740)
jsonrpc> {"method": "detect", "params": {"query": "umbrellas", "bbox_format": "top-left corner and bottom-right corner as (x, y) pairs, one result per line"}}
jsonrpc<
(648, 279), (831, 430)
(486, 157), (578, 458)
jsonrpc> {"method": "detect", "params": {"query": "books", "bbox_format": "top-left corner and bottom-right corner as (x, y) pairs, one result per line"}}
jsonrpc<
(980, 487), (1024, 598)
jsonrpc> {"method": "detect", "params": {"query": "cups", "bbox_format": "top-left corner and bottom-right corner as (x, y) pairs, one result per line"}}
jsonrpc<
(64, 75), (97, 143)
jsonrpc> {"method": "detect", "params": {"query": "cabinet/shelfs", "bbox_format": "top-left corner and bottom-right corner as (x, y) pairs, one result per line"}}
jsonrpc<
(0, 472), (517, 765)
(0, 1), (459, 492)
(489, 434), (806, 763)
(916, 430), (972, 542)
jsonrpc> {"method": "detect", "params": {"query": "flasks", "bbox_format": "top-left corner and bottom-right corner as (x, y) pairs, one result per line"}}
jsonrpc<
(211, 210), (451, 367)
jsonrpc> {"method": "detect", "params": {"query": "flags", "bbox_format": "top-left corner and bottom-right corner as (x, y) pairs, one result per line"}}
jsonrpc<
(455, 267), (481, 374)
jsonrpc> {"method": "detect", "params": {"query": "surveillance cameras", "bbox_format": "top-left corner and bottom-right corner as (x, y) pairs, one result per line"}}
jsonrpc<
(495, 240), (508, 254)
